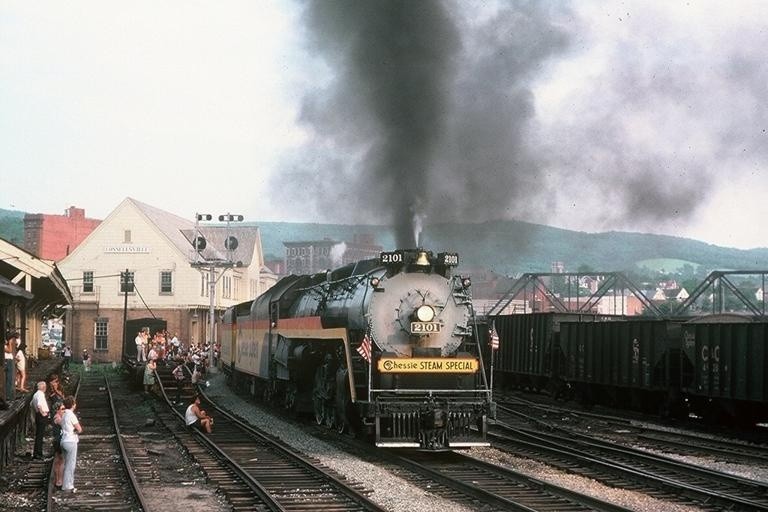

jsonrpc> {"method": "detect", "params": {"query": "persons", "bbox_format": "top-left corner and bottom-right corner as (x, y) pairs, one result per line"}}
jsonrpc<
(134, 325), (222, 434)
(3, 331), (92, 493)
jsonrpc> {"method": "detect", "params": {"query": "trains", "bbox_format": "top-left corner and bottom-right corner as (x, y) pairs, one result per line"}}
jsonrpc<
(216, 246), (491, 454)
(470, 313), (768, 423)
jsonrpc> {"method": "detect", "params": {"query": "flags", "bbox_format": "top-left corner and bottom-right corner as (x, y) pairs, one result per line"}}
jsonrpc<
(355, 316), (372, 364)
(487, 324), (500, 350)
